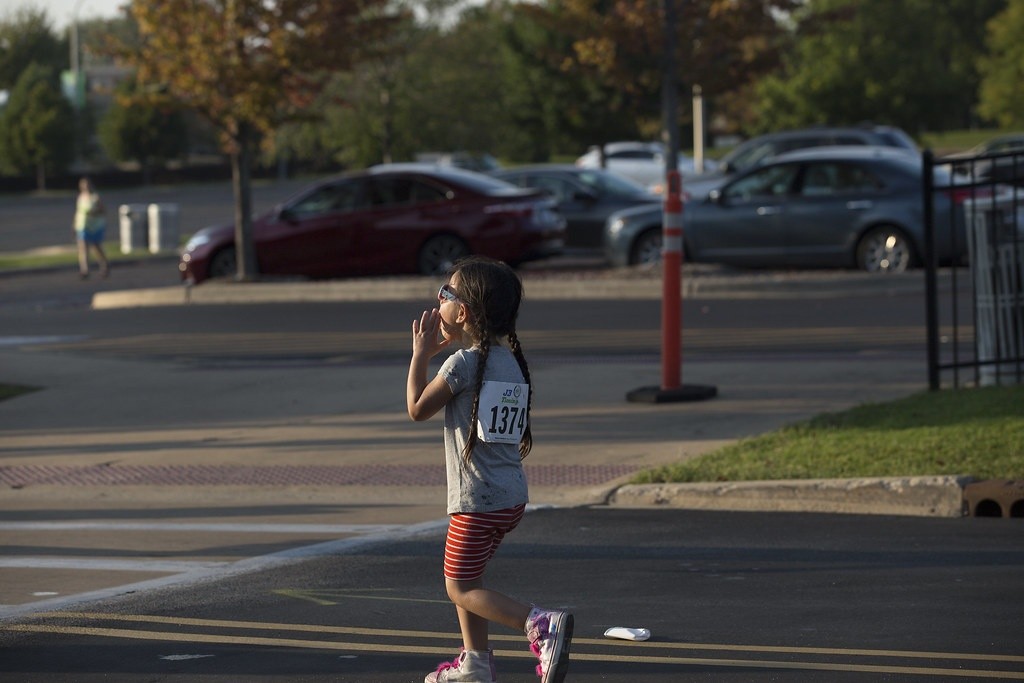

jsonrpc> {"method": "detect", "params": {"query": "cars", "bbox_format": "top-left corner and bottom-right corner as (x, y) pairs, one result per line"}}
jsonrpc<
(651, 127), (921, 193)
(574, 142), (714, 193)
(179, 163), (567, 286)
(602, 144), (1002, 276)
(475, 167), (692, 271)
(934, 136), (1024, 186)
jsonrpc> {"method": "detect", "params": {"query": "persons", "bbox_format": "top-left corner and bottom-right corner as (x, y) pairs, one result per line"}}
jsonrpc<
(71, 179), (110, 279)
(404, 257), (575, 682)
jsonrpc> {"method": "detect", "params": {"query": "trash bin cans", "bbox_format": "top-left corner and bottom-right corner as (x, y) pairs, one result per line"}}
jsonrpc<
(119, 203), (147, 254)
(147, 201), (181, 255)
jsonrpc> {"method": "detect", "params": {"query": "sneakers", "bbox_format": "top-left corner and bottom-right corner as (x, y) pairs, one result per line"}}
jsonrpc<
(424, 647), (497, 683)
(523, 603), (575, 683)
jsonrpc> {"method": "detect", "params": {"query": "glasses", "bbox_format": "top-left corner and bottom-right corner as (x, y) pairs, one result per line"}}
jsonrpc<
(437, 283), (466, 306)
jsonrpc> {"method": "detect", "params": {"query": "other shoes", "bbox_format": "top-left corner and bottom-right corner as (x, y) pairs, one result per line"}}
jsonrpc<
(77, 272), (92, 281)
(98, 267), (109, 280)
